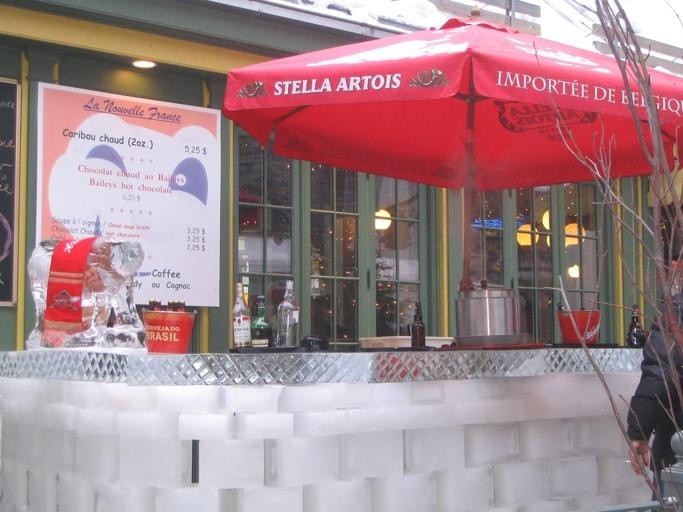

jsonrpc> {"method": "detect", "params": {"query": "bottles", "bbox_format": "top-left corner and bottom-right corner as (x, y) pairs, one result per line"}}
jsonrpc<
(557, 302), (567, 311)
(304, 335), (329, 352)
(233, 281), (301, 350)
(410, 301), (424, 347)
(147, 299), (187, 312)
(628, 305), (646, 348)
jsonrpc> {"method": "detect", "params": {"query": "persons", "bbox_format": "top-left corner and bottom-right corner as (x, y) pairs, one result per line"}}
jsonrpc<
(626, 242), (682, 500)
